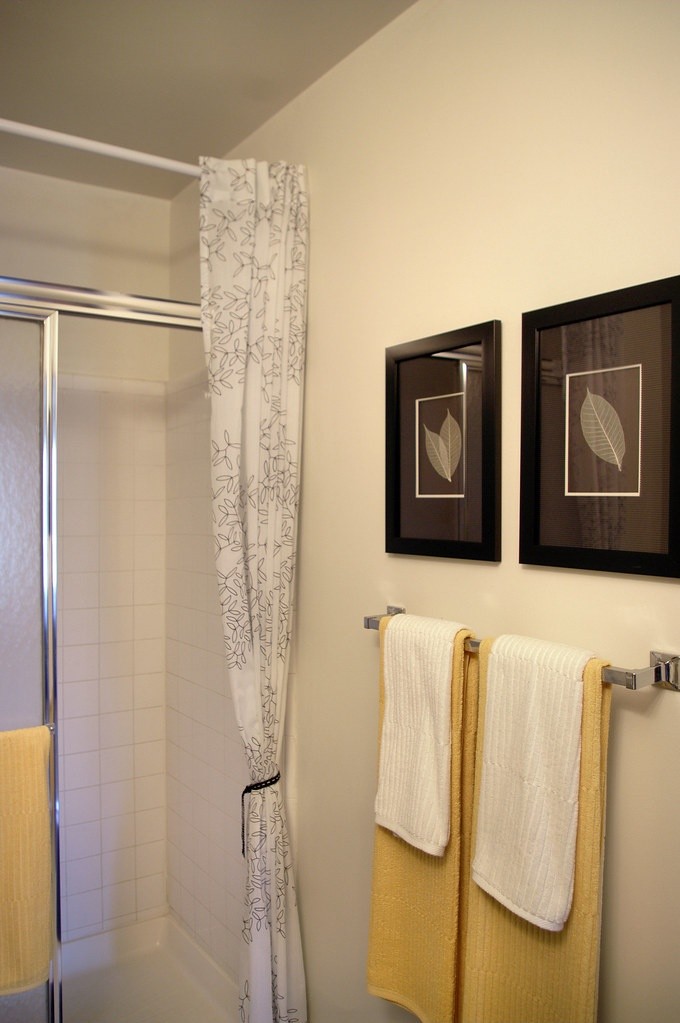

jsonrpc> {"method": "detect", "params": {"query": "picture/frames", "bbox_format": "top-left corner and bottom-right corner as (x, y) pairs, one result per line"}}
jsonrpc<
(385, 321), (501, 562)
(518, 276), (679, 579)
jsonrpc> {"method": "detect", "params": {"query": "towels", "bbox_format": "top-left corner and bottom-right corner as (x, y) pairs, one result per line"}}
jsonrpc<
(0, 724), (53, 995)
(367, 612), (612, 1023)
